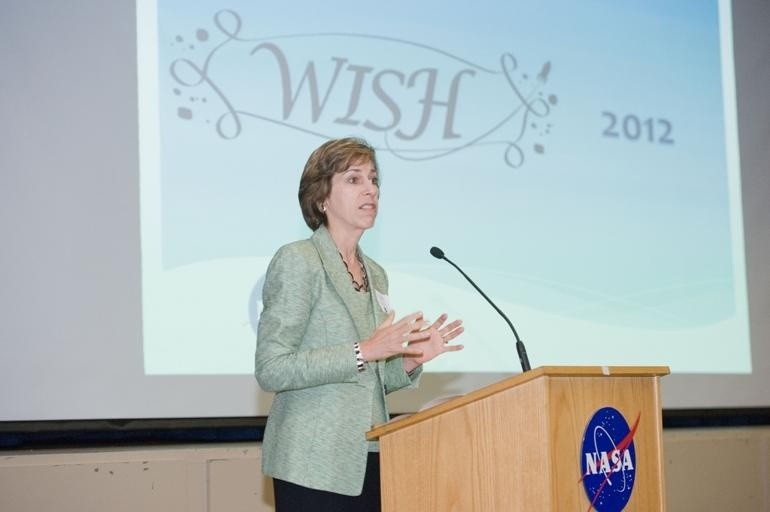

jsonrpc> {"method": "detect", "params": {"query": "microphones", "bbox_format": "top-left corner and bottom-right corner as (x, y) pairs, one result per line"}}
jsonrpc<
(430, 246), (531, 373)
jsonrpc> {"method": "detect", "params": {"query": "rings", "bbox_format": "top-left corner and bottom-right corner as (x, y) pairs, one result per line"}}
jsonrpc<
(443, 335), (449, 344)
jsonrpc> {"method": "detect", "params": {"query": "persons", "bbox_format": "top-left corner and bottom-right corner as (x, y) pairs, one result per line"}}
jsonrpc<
(253, 138), (465, 512)
